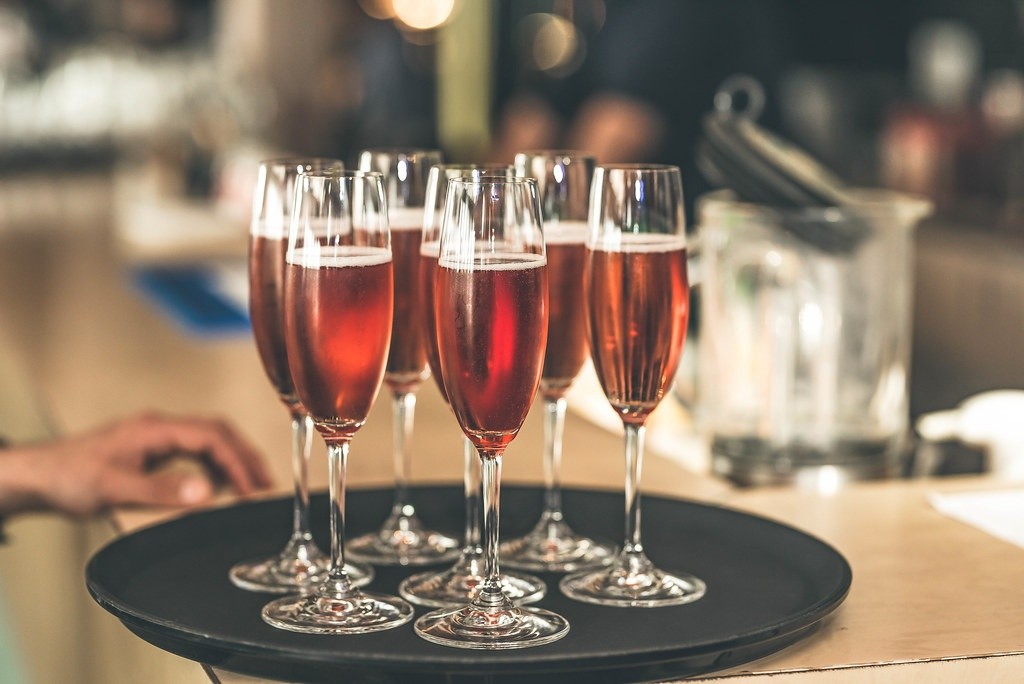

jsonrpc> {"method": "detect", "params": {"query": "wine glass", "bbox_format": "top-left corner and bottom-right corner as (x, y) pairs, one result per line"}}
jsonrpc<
(244, 146), (707, 650)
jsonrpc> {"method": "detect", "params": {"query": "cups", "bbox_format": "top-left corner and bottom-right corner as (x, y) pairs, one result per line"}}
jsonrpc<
(697, 197), (933, 494)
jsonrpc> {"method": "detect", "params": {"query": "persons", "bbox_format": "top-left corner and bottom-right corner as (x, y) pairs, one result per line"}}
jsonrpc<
(0, 409), (275, 544)
